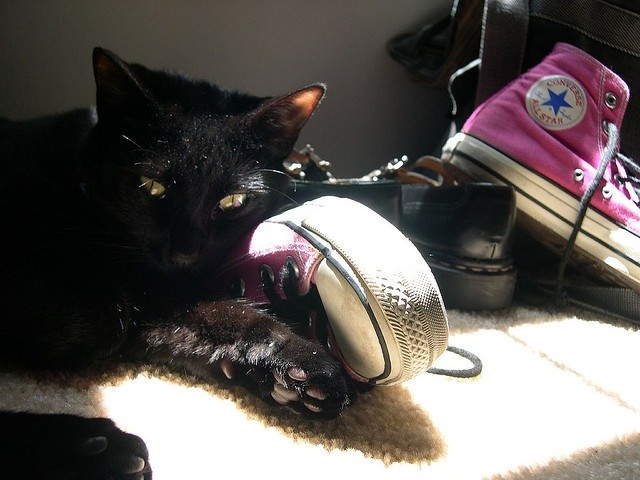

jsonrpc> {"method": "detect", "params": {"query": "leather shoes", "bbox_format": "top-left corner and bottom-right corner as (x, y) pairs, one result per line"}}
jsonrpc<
(276, 144), (519, 311)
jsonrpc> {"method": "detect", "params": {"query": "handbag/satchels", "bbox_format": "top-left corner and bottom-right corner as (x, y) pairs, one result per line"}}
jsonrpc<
(386, 1), (640, 152)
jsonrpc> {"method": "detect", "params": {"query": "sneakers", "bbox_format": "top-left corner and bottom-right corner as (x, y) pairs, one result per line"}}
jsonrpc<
(441, 43), (640, 293)
(215, 196), (449, 387)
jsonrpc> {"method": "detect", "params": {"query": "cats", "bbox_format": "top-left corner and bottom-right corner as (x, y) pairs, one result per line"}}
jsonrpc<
(0, 46), (348, 480)
